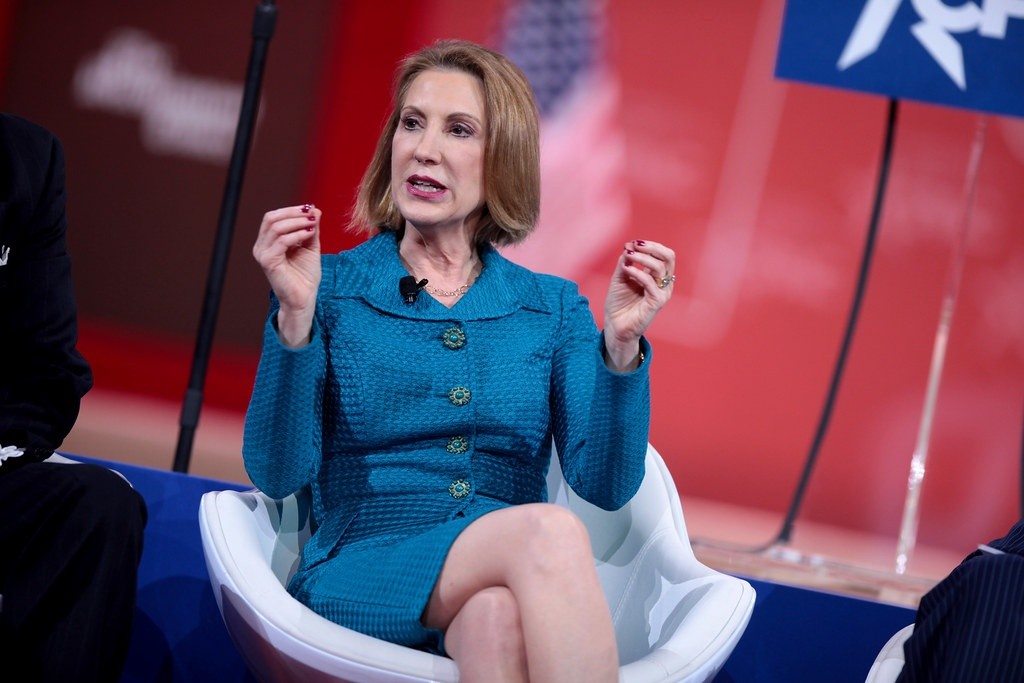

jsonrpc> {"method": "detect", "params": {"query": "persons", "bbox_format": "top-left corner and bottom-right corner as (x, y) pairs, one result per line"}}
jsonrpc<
(242, 36), (677, 683)
(894, 519), (1024, 683)
(0, 111), (163, 683)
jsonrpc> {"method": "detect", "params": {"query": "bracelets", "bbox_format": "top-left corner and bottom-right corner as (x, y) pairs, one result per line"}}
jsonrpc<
(638, 348), (646, 365)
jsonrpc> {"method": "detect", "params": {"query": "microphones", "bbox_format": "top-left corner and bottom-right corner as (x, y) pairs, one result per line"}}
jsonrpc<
(399, 276), (420, 304)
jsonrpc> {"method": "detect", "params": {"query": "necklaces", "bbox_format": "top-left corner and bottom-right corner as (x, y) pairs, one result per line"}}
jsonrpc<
(397, 241), (482, 296)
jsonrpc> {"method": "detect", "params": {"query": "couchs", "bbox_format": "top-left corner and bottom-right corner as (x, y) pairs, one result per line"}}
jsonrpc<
(198, 439), (756, 683)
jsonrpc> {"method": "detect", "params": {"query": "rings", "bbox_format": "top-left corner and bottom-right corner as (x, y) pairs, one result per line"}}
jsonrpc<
(655, 271), (675, 288)
